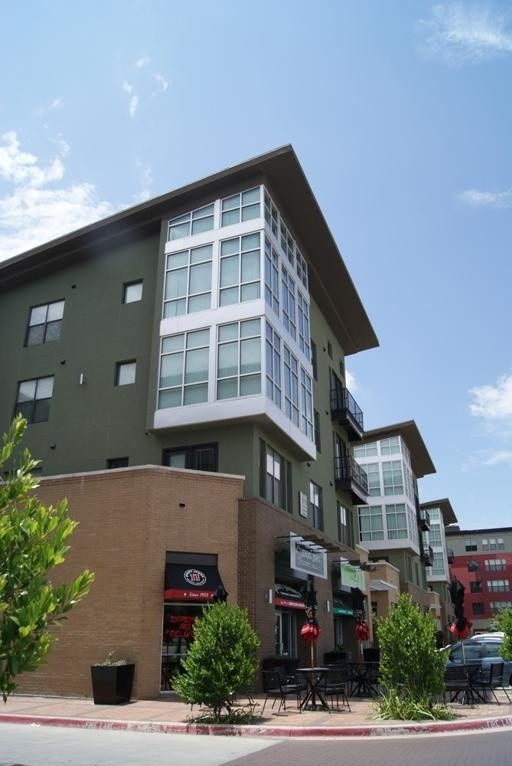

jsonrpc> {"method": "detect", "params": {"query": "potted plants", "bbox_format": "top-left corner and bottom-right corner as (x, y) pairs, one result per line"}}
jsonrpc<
(91, 649), (136, 705)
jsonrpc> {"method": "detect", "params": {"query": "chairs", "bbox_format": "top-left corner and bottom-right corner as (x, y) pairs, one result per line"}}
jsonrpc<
(261, 660), (512, 717)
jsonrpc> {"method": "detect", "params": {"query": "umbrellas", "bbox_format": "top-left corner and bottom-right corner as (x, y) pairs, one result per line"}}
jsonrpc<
(350, 586), (370, 693)
(298, 579), (323, 705)
(214, 583), (227, 603)
(446, 573), (474, 665)
(435, 630), (445, 650)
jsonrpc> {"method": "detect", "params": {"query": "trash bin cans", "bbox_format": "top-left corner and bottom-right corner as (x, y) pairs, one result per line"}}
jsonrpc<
(90, 663), (135, 705)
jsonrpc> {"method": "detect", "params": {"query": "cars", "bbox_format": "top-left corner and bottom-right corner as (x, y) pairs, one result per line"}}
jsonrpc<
(434, 631), (512, 693)
